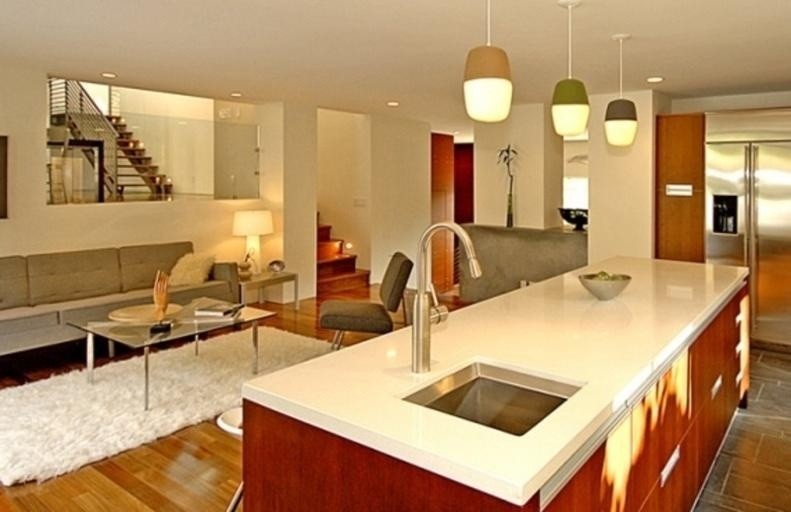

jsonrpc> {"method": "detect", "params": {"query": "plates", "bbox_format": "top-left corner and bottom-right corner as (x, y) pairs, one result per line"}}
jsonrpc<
(108, 318), (183, 338)
(106, 303), (184, 324)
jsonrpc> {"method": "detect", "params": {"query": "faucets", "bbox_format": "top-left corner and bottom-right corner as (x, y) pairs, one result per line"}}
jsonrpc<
(411, 221), (483, 373)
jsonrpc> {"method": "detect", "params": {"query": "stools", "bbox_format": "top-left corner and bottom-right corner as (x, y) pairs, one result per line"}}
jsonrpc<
(215, 404), (246, 512)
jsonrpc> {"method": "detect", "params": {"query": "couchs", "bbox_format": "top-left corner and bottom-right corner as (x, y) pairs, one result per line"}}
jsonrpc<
(0, 242), (239, 367)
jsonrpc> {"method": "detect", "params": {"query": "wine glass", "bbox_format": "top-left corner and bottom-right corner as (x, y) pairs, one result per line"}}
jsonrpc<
(558, 208), (588, 232)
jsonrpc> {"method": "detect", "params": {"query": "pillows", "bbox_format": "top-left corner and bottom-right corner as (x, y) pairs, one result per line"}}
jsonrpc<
(169, 253), (216, 287)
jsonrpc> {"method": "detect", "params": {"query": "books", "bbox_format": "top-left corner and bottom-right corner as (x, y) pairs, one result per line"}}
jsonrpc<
(165, 296), (245, 324)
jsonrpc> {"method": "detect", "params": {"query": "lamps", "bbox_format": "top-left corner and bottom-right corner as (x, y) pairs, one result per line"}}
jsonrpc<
(233, 210), (273, 275)
(461, 0), (514, 124)
(605, 34), (638, 147)
(551, 0), (590, 137)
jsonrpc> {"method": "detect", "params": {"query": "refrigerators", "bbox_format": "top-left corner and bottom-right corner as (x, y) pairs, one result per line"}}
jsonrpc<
(704, 107), (791, 351)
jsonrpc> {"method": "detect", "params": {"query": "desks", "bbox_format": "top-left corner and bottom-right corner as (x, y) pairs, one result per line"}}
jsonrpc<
(237, 268), (300, 312)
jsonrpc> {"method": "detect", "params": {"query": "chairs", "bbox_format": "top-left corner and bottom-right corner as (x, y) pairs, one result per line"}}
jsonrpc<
(317, 251), (414, 353)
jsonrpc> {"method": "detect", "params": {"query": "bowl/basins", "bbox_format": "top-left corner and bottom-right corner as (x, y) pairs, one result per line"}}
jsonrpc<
(580, 273), (633, 301)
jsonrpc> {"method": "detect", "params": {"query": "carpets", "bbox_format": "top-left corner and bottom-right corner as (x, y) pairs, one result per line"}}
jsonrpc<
(0, 326), (349, 487)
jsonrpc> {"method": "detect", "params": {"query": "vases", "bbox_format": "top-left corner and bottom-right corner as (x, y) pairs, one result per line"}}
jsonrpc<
(506, 194), (513, 228)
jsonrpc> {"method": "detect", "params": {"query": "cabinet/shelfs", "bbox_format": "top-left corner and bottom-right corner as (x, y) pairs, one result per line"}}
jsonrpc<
(655, 112), (704, 263)
(522, 273), (749, 511)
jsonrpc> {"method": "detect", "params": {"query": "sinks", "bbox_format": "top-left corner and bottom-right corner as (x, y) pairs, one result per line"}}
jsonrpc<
(394, 353), (588, 436)
